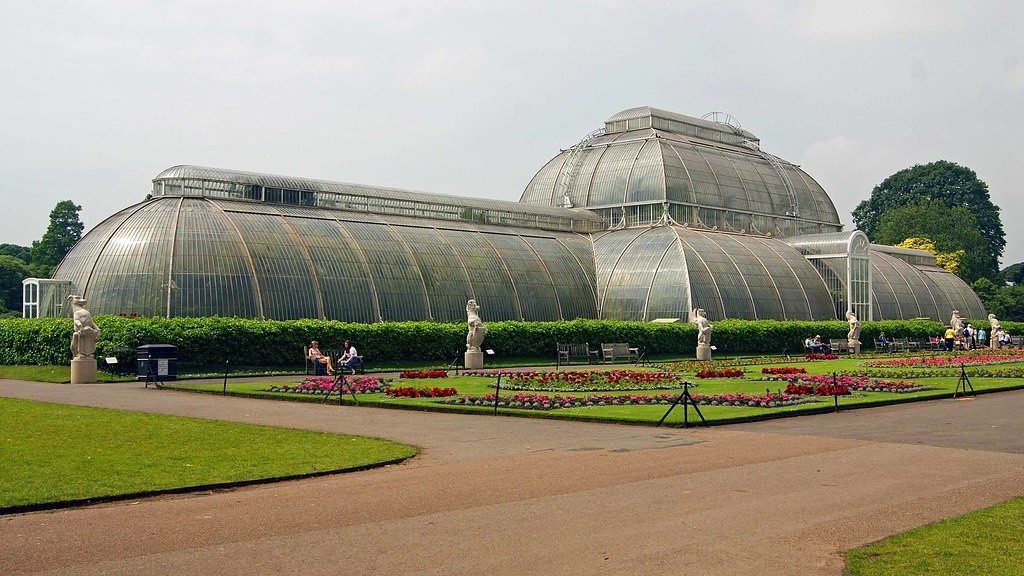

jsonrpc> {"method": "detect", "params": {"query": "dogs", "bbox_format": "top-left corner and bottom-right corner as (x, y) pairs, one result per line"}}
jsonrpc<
(695, 309), (712, 345)
(846, 311), (862, 342)
(68, 295), (100, 357)
(466, 299), (487, 350)
(950, 310), (965, 338)
(988, 314), (1002, 337)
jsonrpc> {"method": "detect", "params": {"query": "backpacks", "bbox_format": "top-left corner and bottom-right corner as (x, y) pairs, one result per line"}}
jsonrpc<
(962, 329), (969, 338)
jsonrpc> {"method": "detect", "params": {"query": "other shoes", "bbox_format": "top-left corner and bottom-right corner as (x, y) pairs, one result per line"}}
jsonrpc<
(352, 370), (355, 375)
(330, 369), (335, 372)
(326, 372), (333, 376)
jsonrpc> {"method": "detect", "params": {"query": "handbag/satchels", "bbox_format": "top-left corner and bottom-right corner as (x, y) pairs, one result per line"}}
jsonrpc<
(315, 364), (327, 375)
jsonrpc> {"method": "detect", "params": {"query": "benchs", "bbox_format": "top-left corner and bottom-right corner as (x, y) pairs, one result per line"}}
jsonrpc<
(601, 343), (639, 365)
(303, 346), (364, 374)
(874, 338), (948, 350)
(802, 338), (855, 356)
(557, 342), (599, 365)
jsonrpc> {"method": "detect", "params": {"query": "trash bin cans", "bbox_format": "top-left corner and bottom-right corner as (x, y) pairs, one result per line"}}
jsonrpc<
(136, 344), (178, 382)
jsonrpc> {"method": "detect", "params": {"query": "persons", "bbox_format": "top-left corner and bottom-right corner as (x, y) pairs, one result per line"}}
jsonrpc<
(338, 340), (358, 375)
(933, 324), (1011, 351)
(877, 332), (892, 353)
(805, 334), (831, 354)
(309, 341), (336, 376)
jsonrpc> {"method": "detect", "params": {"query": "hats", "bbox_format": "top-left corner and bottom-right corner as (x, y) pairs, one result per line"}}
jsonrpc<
(968, 324), (971, 327)
(816, 335), (820, 338)
